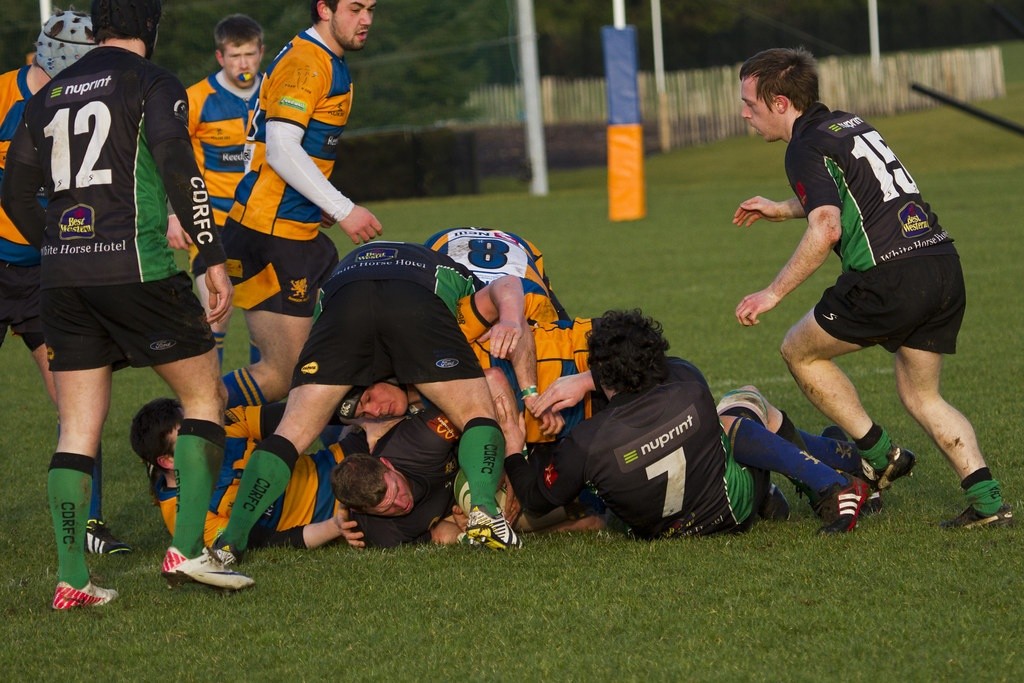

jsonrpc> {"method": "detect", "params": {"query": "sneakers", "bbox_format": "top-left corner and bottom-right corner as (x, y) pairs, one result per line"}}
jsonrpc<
(855, 444), (917, 492)
(211, 537), (244, 567)
(161, 546), (254, 590)
(941, 506), (1016, 531)
(84, 519), (132, 555)
(50, 582), (120, 610)
(815, 470), (870, 536)
(464, 504), (524, 553)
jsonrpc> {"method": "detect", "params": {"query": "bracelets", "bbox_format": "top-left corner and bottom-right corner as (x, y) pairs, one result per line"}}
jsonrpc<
(521, 386), (537, 400)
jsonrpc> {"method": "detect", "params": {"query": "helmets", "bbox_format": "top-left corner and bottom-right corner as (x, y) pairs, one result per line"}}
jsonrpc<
(34, 11), (98, 79)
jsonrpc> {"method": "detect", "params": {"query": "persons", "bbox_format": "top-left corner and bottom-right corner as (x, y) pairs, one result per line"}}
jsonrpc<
(732, 47), (1014, 531)
(128, 226), (884, 566)
(166, 15), (264, 365)
(0, 0), (254, 610)
(224, 0), (385, 407)
(0, 11), (134, 556)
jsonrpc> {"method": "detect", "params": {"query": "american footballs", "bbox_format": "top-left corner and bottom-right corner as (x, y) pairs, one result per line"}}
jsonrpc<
(451, 466), (510, 520)
